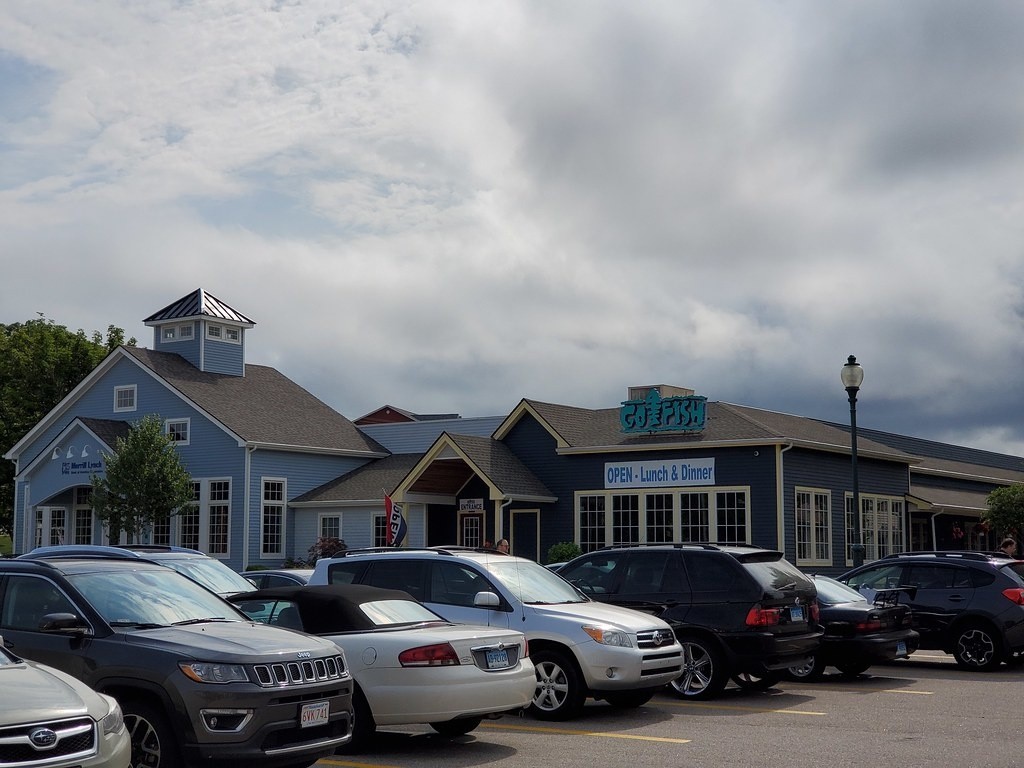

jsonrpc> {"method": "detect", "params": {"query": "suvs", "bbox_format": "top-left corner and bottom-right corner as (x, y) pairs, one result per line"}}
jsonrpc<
(306, 545), (684, 721)
(553, 541), (827, 702)
(14, 544), (293, 626)
(0, 555), (354, 768)
(828, 550), (1023, 672)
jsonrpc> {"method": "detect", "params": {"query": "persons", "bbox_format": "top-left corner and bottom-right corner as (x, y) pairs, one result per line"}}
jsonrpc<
(484, 538), (494, 553)
(495, 539), (509, 554)
(999, 539), (1017, 556)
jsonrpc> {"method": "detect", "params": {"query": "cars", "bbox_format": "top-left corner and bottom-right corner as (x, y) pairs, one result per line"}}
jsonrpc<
(0, 636), (131, 768)
(543, 562), (616, 596)
(236, 569), (316, 589)
(777, 572), (920, 684)
(222, 584), (535, 757)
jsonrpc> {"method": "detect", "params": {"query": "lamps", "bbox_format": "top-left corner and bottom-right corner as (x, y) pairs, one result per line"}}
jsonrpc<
(67, 445), (78, 459)
(82, 445), (95, 458)
(52, 447), (66, 461)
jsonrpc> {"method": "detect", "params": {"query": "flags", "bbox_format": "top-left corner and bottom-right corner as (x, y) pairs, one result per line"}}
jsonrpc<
(385, 495), (408, 547)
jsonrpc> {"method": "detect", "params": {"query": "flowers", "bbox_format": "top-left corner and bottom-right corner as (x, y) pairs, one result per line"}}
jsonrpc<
(951, 527), (965, 538)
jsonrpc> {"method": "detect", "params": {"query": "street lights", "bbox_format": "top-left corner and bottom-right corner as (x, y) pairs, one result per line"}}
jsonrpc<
(842, 354), (867, 570)
